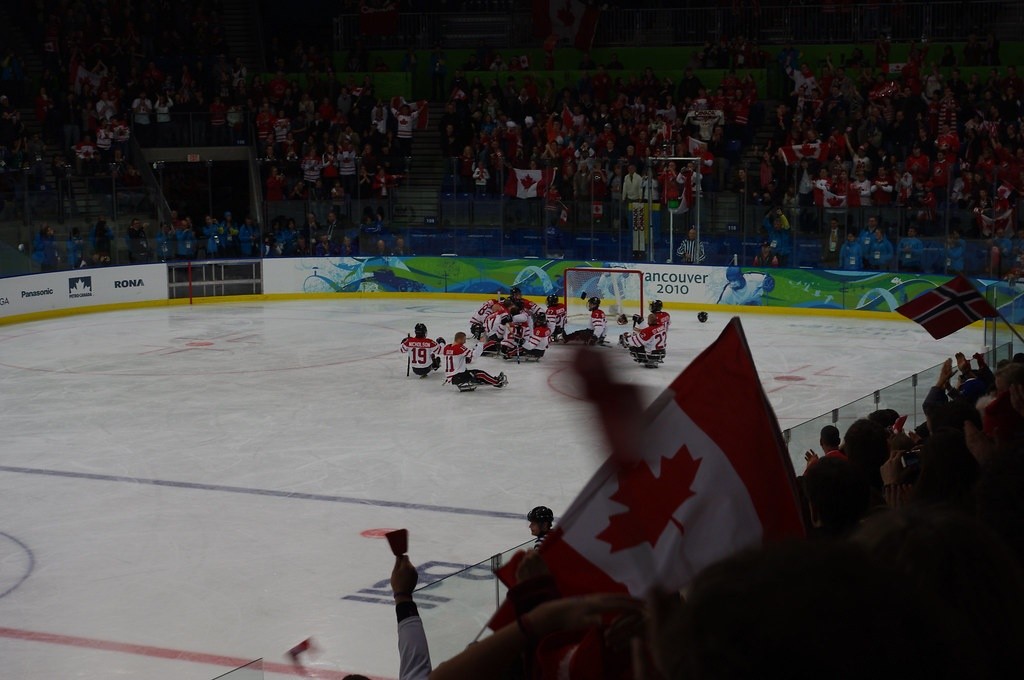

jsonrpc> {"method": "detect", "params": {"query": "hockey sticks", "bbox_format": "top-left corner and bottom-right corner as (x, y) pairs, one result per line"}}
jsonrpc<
(496, 290), (501, 304)
(405, 333), (411, 377)
(441, 344), (477, 386)
(517, 346), (521, 364)
(506, 323), (516, 335)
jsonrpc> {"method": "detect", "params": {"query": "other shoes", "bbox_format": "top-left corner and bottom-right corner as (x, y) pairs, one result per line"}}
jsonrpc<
(495, 372), (507, 387)
(561, 329), (569, 343)
(434, 356), (440, 370)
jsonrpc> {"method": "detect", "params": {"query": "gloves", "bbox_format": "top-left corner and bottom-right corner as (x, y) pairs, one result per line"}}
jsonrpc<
(499, 314), (513, 325)
(762, 277), (774, 291)
(436, 337), (445, 345)
(401, 337), (412, 344)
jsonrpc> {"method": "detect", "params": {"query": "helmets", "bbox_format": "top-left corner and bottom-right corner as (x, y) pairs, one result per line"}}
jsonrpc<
(534, 312), (547, 326)
(547, 294), (557, 307)
(588, 297), (601, 312)
(726, 267), (746, 290)
(650, 300), (662, 313)
(510, 287), (520, 296)
(527, 507), (554, 521)
(414, 323), (427, 334)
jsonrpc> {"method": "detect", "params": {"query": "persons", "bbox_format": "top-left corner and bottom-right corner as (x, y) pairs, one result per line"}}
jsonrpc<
(399, 286), (669, 393)
(343, 351), (1024, 680)
(0, 0), (1024, 283)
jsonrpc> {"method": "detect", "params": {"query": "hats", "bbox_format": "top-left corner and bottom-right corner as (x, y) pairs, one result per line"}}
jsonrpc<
(605, 123), (611, 129)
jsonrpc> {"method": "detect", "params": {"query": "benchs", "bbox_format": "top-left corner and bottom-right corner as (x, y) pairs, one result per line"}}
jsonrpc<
(0, 0), (791, 243)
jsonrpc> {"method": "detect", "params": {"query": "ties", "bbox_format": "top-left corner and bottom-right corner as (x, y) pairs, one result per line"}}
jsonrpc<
(831, 231), (836, 243)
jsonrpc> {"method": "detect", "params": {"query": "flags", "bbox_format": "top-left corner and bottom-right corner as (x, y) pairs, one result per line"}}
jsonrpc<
(393, 96), (429, 131)
(487, 317), (811, 636)
(894, 276), (1002, 340)
(504, 167), (557, 199)
(778, 142), (829, 165)
(812, 186), (861, 214)
